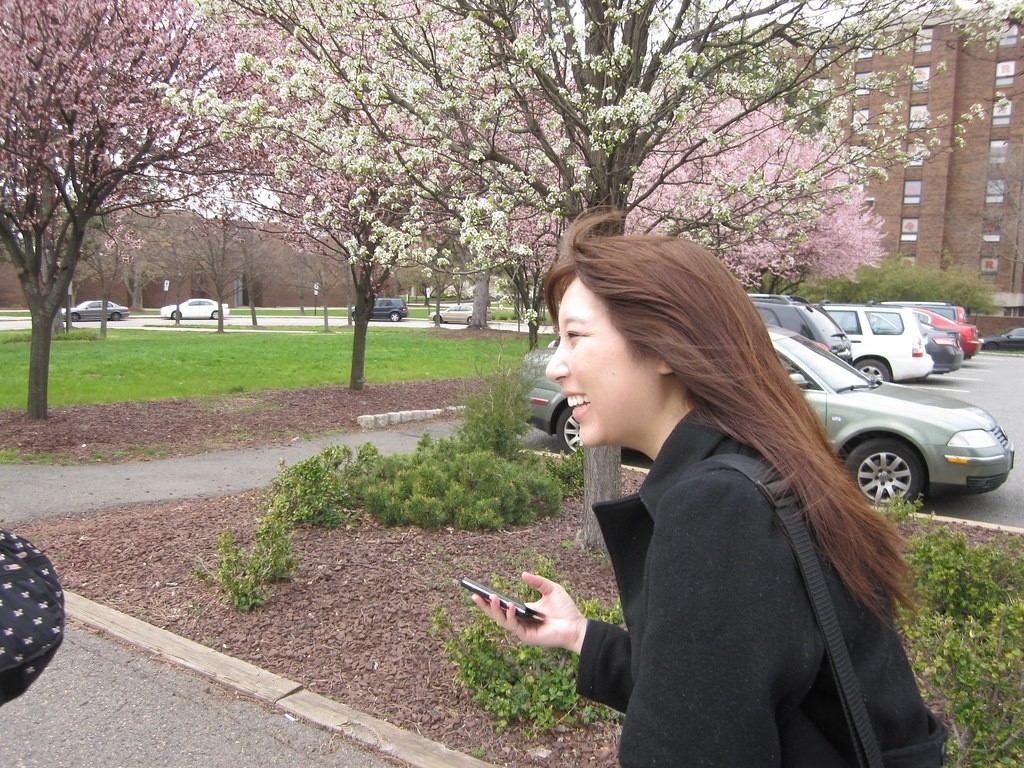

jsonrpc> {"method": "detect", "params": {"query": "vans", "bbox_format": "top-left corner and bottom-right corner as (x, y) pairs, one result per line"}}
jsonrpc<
(876, 298), (968, 324)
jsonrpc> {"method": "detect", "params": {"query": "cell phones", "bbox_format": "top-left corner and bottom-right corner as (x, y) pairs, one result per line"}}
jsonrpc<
(461, 577), (546, 623)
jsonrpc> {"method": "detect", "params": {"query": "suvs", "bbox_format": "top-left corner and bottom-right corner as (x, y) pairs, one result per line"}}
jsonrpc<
(811, 299), (935, 383)
(351, 297), (410, 322)
(744, 292), (854, 368)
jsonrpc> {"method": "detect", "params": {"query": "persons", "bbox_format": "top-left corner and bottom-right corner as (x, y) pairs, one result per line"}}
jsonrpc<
(471, 206), (949, 768)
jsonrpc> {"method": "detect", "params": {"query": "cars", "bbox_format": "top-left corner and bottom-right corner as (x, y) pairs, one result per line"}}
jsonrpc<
(62, 300), (129, 322)
(908, 306), (983, 359)
(977, 325), (1024, 350)
(509, 318), (1016, 508)
(920, 320), (964, 376)
(428, 304), (493, 325)
(161, 298), (230, 320)
